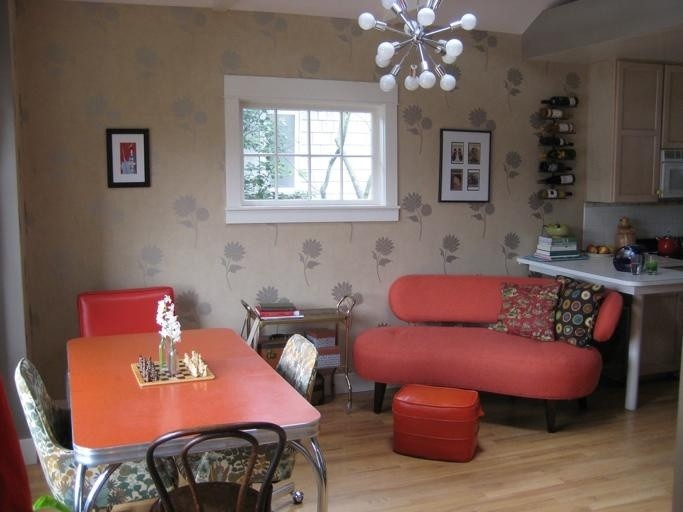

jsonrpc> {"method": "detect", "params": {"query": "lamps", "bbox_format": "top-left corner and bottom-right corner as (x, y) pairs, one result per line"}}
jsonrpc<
(351, 0), (481, 94)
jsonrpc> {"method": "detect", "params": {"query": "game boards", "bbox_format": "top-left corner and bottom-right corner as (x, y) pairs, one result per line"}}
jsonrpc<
(131, 359), (215, 387)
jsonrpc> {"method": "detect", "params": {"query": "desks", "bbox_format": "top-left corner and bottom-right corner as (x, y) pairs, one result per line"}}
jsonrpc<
(513, 249), (682, 416)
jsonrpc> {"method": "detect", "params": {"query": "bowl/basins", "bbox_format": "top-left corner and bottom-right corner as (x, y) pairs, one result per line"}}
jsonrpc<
(545, 224), (569, 237)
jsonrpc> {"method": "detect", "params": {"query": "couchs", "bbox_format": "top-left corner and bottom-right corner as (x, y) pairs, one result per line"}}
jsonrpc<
(346, 271), (623, 434)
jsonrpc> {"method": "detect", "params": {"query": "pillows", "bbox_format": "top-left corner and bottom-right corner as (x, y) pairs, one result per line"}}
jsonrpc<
(552, 272), (603, 350)
(484, 281), (562, 344)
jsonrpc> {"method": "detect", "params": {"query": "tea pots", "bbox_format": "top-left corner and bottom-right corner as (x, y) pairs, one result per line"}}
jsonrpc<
(655, 235), (678, 255)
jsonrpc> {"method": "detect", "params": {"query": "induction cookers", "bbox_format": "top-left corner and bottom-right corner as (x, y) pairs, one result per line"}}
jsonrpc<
(648, 251), (683, 261)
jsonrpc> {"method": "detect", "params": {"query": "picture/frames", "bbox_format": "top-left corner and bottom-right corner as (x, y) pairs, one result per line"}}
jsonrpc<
(436, 127), (494, 205)
(105, 127), (152, 189)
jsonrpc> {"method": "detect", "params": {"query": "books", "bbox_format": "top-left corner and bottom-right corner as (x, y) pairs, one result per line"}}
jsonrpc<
(255, 303), (305, 321)
(524, 235), (587, 262)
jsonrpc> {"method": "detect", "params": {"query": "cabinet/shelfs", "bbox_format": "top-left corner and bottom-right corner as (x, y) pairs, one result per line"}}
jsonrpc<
(239, 294), (357, 416)
(580, 52), (663, 210)
(657, 60), (682, 154)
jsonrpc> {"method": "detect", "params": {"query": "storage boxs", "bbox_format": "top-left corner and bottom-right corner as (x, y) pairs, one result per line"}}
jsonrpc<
(304, 326), (337, 348)
(310, 344), (342, 370)
(255, 333), (291, 367)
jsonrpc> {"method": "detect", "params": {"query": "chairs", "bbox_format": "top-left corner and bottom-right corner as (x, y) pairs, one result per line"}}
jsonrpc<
(71, 284), (175, 338)
(10, 352), (183, 511)
(175, 331), (323, 506)
(144, 419), (289, 512)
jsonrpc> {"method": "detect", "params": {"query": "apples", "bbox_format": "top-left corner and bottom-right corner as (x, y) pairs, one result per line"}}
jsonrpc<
(588, 243), (609, 255)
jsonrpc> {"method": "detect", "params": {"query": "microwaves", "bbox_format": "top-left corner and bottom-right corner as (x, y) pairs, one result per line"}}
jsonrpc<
(659, 147), (683, 201)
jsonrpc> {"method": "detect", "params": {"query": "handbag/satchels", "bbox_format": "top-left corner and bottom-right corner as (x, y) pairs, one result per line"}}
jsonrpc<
(257, 334), (292, 369)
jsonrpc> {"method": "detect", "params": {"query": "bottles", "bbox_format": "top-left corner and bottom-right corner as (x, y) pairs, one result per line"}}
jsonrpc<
(533, 95), (578, 200)
(615, 217), (637, 252)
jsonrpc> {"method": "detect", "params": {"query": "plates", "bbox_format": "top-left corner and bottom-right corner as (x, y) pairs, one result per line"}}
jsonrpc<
(586, 253), (614, 258)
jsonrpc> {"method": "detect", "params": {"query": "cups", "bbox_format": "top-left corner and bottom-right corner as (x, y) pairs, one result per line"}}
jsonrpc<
(644, 255), (657, 274)
(630, 255), (642, 275)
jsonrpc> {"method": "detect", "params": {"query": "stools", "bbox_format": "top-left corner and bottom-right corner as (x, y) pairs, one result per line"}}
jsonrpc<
(388, 380), (488, 463)
